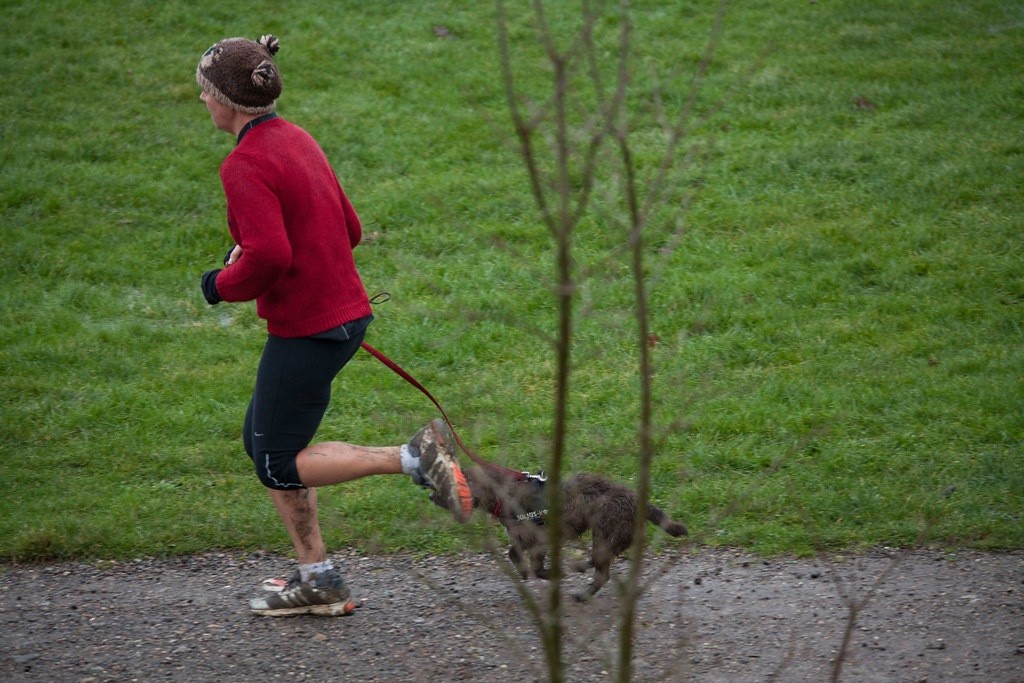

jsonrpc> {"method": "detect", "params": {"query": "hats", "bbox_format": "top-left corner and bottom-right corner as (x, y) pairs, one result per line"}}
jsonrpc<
(195, 35), (283, 114)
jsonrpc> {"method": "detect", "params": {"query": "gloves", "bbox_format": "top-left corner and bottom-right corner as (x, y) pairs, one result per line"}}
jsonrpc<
(201, 268), (223, 306)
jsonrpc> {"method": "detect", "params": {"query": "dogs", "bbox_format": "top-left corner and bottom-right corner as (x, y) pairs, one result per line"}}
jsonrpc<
(429, 463), (688, 601)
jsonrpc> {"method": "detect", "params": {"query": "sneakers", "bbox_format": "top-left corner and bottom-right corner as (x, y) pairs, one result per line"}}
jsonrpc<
(408, 418), (474, 522)
(250, 566), (353, 616)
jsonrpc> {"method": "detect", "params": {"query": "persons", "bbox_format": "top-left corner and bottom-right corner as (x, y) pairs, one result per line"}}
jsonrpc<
(195, 34), (474, 617)
